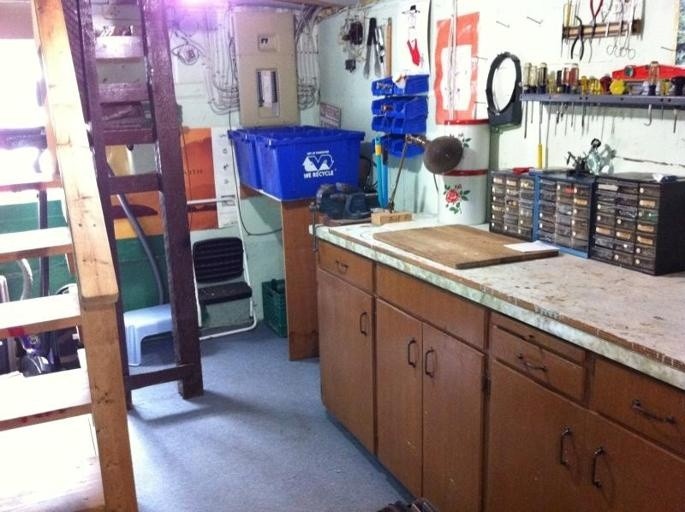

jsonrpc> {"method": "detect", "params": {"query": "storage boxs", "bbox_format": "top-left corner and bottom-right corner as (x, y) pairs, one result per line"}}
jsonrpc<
(230, 123), (367, 202)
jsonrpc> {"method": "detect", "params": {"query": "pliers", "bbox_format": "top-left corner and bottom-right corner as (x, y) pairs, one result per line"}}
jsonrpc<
(590, 0), (602, 39)
(571, 16), (585, 60)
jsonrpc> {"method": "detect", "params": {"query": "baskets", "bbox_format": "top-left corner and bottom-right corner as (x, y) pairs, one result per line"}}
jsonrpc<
(262, 279), (287, 338)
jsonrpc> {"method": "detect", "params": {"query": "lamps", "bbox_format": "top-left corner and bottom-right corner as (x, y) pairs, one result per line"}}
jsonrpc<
(381, 133), (462, 213)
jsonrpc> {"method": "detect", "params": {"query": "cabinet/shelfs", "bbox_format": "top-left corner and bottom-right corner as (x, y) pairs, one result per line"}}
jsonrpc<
(373, 262), (489, 512)
(317, 241), (373, 460)
(488, 310), (685, 512)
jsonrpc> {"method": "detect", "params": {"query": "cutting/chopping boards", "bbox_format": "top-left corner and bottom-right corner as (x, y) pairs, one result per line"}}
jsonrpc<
(372, 224), (559, 269)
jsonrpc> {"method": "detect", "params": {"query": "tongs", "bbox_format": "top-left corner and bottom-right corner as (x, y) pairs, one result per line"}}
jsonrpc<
(362, 17), (383, 79)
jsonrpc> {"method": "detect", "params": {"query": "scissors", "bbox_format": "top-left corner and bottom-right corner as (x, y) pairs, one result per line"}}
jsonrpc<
(607, 4), (639, 59)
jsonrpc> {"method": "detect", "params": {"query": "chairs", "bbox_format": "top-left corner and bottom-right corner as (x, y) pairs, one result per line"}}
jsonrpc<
(191, 237), (254, 327)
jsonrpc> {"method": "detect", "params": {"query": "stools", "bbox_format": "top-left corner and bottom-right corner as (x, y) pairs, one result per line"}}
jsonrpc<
(122, 303), (173, 367)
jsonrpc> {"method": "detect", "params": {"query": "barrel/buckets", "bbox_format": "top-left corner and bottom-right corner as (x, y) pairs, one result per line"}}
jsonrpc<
(436, 174), (488, 226)
(443, 119), (490, 174)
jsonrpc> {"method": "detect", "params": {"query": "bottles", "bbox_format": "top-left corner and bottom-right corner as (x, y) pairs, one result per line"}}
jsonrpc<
(521, 60), (548, 88)
(661, 76), (670, 96)
(562, 61), (579, 91)
(648, 60), (659, 95)
(581, 75), (587, 97)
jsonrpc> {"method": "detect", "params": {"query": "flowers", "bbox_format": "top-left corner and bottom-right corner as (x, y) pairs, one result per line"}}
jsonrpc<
(444, 183), (470, 215)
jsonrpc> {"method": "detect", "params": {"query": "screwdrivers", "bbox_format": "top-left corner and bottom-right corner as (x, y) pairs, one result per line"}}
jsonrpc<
(647, 61), (685, 133)
(521, 61), (603, 138)
